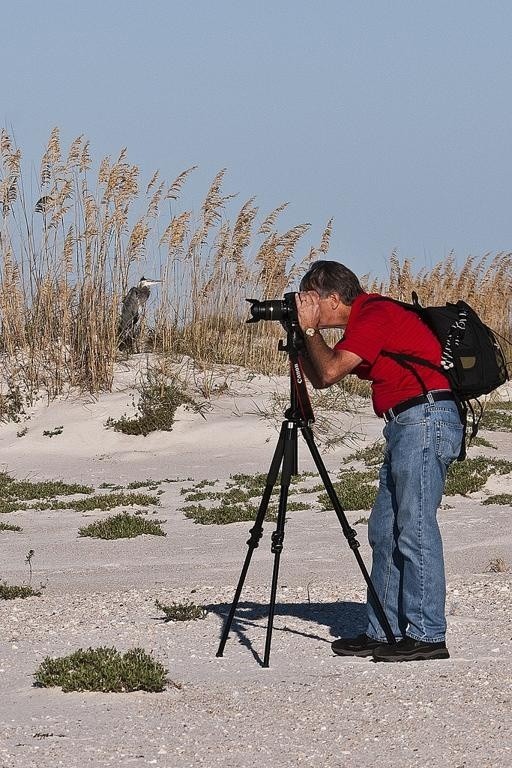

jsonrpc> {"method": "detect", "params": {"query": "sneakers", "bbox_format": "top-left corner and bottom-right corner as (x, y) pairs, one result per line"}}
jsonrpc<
(332, 633), (386, 655)
(374, 636), (450, 662)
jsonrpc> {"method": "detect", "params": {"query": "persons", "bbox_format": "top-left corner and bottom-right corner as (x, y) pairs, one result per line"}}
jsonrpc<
(288, 261), (464, 662)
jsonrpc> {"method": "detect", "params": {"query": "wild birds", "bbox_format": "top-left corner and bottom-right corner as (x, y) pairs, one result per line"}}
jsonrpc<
(117, 276), (166, 352)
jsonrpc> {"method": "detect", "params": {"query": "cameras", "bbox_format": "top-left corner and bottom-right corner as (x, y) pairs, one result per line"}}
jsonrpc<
(246, 291), (299, 323)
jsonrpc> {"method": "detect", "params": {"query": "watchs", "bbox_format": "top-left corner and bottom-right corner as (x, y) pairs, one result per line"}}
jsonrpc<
(303, 327), (320, 338)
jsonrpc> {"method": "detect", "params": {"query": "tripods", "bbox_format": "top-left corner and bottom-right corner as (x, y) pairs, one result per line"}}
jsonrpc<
(216, 319), (396, 669)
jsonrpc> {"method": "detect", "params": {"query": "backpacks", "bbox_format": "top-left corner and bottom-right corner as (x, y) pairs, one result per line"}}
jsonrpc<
(363, 295), (509, 401)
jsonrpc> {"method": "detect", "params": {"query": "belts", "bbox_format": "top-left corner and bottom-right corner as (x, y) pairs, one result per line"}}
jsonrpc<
(383, 392), (459, 421)
